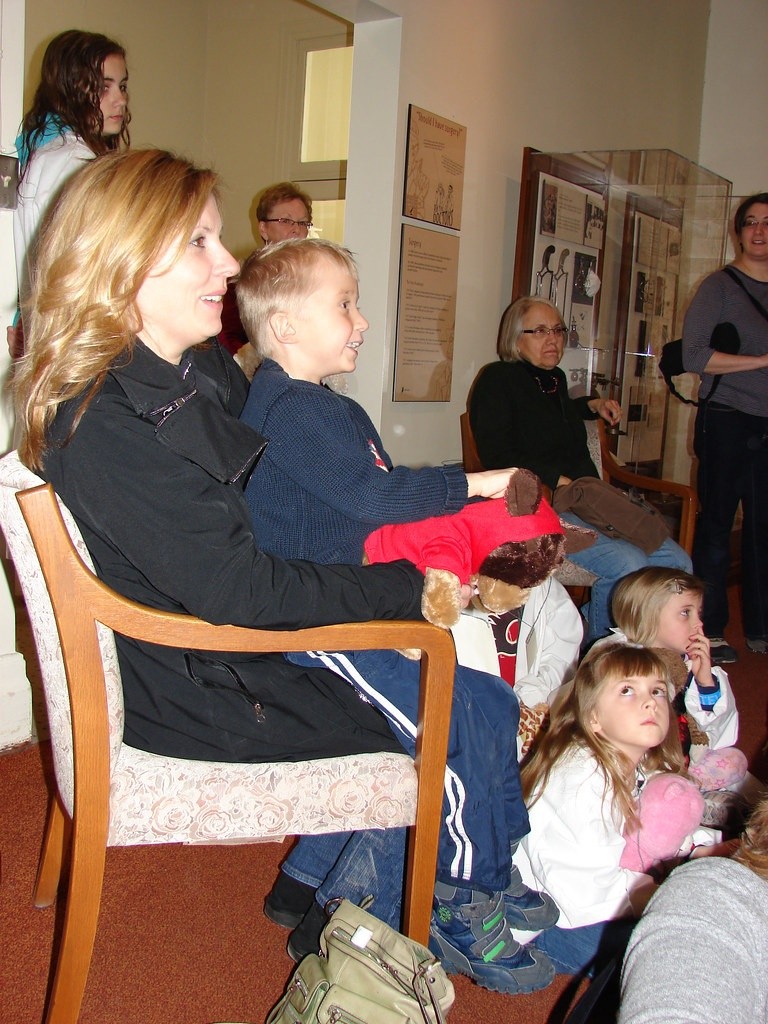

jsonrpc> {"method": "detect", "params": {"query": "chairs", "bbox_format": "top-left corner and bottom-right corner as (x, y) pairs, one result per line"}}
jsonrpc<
(461, 405), (695, 618)
(0, 451), (456, 1024)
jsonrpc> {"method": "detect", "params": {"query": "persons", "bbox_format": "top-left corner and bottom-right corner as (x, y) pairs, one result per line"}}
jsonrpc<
(216, 180), (313, 357)
(467, 296), (693, 656)
(617, 790), (768, 1024)
(682, 192), (768, 663)
(450, 576), (583, 769)
(611, 566), (768, 805)
(506, 640), (742, 980)
(0, 141), (426, 965)
(237, 236), (559, 995)
(0, 28), (132, 358)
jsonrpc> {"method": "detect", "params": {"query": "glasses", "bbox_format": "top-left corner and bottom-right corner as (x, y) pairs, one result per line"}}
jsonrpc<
(264, 218), (313, 230)
(522, 326), (568, 337)
(744, 220), (768, 230)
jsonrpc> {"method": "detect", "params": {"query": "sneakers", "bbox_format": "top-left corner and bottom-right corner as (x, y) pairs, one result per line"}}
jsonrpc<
(428, 882), (555, 994)
(708, 637), (737, 663)
(746, 636), (768, 654)
(496, 866), (560, 932)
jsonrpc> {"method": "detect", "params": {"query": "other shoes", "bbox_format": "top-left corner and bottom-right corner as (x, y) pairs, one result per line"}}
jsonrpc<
(287, 900), (334, 964)
(263, 869), (319, 928)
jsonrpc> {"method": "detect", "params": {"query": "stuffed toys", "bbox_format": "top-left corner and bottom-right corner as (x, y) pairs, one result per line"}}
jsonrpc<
(361, 468), (565, 660)
(235, 342), (349, 395)
(619, 747), (748, 873)
(516, 701), (550, 756)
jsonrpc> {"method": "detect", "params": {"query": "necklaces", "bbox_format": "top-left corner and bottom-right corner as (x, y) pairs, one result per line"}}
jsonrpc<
(535, 375), (558, 393)
(508, 577), (552, 644)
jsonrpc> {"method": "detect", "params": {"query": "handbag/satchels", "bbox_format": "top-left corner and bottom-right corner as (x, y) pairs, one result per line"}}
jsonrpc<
(552, 476), (674, 556)
(661, 322), (740, 378)
(266, 898), (455, 1024)
